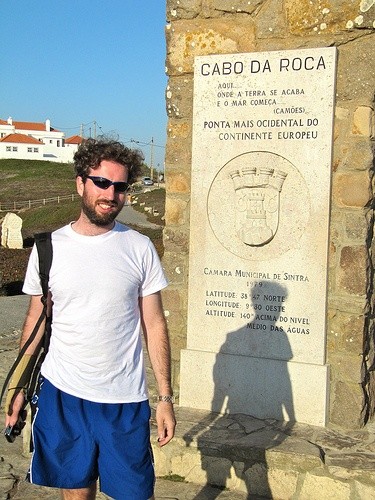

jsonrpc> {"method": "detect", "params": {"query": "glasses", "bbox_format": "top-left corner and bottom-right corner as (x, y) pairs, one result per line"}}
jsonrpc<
(85, 174), (128, 192)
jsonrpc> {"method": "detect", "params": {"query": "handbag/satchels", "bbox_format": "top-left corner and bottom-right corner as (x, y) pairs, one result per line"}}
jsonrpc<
(23, 401), (36, 459)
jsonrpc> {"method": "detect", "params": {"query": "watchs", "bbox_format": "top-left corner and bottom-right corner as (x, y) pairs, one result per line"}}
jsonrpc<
(156, 395), (174, 404)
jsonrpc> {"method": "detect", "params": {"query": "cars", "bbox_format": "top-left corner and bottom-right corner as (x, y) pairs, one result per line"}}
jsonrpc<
(142, 177), (153, 185)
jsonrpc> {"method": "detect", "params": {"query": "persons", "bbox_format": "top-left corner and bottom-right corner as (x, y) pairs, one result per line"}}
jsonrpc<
(7, 135), (176, 500)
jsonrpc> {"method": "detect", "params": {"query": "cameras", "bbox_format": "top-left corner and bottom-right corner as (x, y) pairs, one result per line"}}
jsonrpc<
(4, 414), (24, 443)
